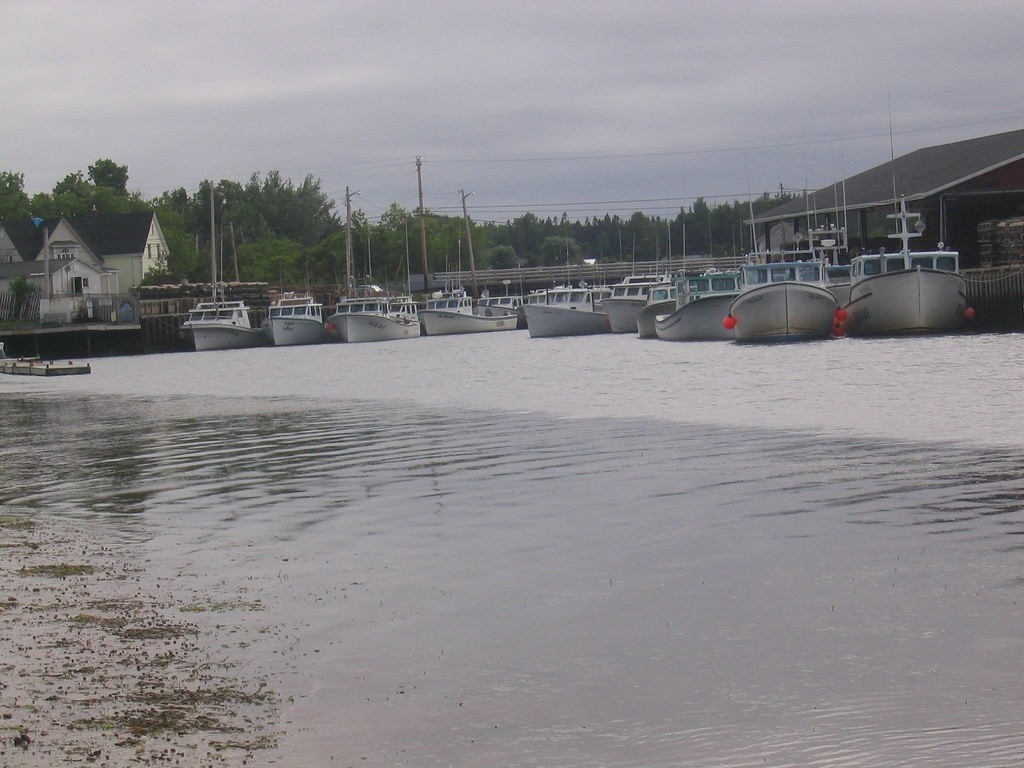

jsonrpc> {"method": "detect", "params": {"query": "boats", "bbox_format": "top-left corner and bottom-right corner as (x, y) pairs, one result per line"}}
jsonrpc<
(841, 107), (967, 333)
(725, 158), (840, 343)
(182, 210), (741, 351)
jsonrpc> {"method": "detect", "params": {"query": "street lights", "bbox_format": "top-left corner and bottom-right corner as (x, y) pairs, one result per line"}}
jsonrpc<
(463, 191), (478, 298)
(346, 191), (362, 234)
